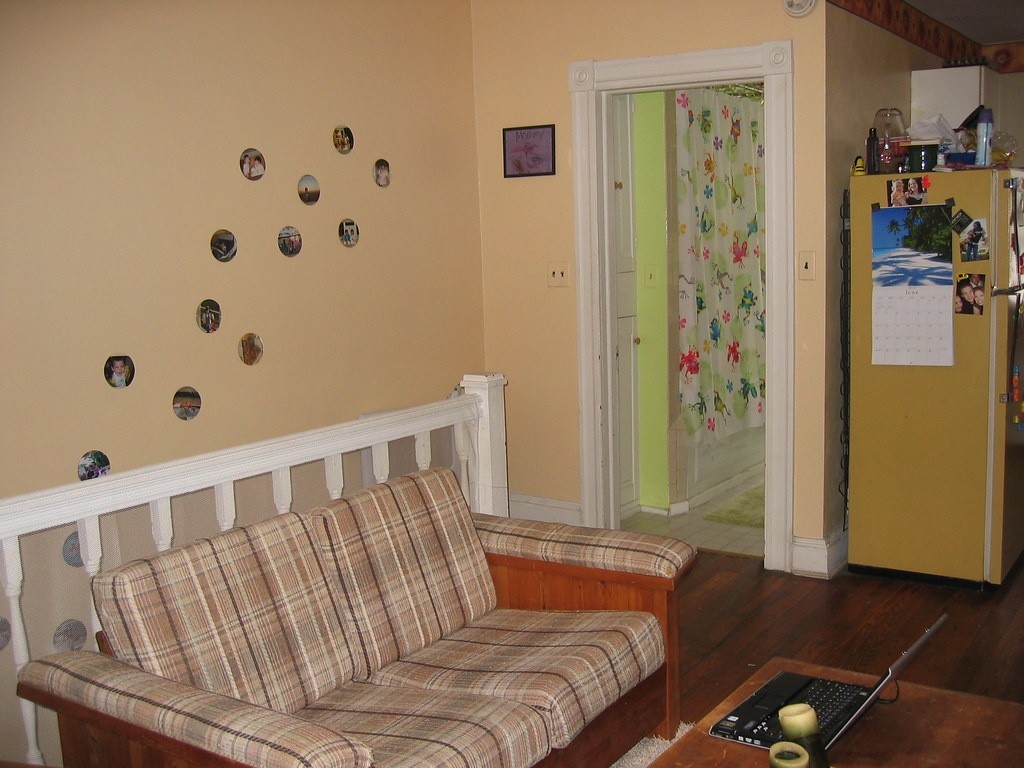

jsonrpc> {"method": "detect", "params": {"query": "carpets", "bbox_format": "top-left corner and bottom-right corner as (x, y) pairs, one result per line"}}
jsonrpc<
(609, 718), (696, 768)
(703, 481), (764, 527)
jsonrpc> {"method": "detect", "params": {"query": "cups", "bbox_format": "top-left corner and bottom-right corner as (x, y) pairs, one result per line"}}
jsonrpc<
(907, 144), (939, 172)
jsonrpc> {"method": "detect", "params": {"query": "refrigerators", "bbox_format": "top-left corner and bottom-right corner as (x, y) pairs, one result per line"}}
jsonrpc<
(849, 168), (1024, 600)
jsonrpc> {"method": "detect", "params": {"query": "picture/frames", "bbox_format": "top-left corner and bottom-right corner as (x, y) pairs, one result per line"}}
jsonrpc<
(503, 124), (556, 178)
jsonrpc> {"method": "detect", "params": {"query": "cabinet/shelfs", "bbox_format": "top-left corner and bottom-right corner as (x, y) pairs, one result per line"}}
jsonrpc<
(910, 66), (1024, 166)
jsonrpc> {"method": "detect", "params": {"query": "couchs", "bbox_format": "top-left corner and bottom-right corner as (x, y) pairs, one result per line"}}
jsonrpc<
(17, 466), (705, 768)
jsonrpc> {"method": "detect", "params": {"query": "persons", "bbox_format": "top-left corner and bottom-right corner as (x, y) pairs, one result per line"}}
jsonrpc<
(242, 340), (253, 365)
(963, 221), (987, 262)
(243, 155), (264, 179)
(344, 229), (357, 245)
(203, 305), (212, 333)
(107, 356), (131, 388)
(891, 178), (926, 207)
(88, 462), (102, 478)
(954, 274), (984, 315)
(335, 129), (350, 149)
(378, 165), (388, 187)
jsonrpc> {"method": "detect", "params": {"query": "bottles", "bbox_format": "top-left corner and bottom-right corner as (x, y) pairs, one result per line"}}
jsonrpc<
(881, 128), (894, 174)
(866, 128), (880, 175)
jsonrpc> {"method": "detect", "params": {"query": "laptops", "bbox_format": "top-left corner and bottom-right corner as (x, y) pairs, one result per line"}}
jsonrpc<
(707, 612), (950, 756)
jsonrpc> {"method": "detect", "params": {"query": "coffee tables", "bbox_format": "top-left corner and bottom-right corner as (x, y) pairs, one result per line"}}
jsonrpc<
(647, 656), (1024, 768)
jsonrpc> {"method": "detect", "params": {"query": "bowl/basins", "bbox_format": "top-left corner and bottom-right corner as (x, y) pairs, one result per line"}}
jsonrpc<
(943, 151), (977, 167)
